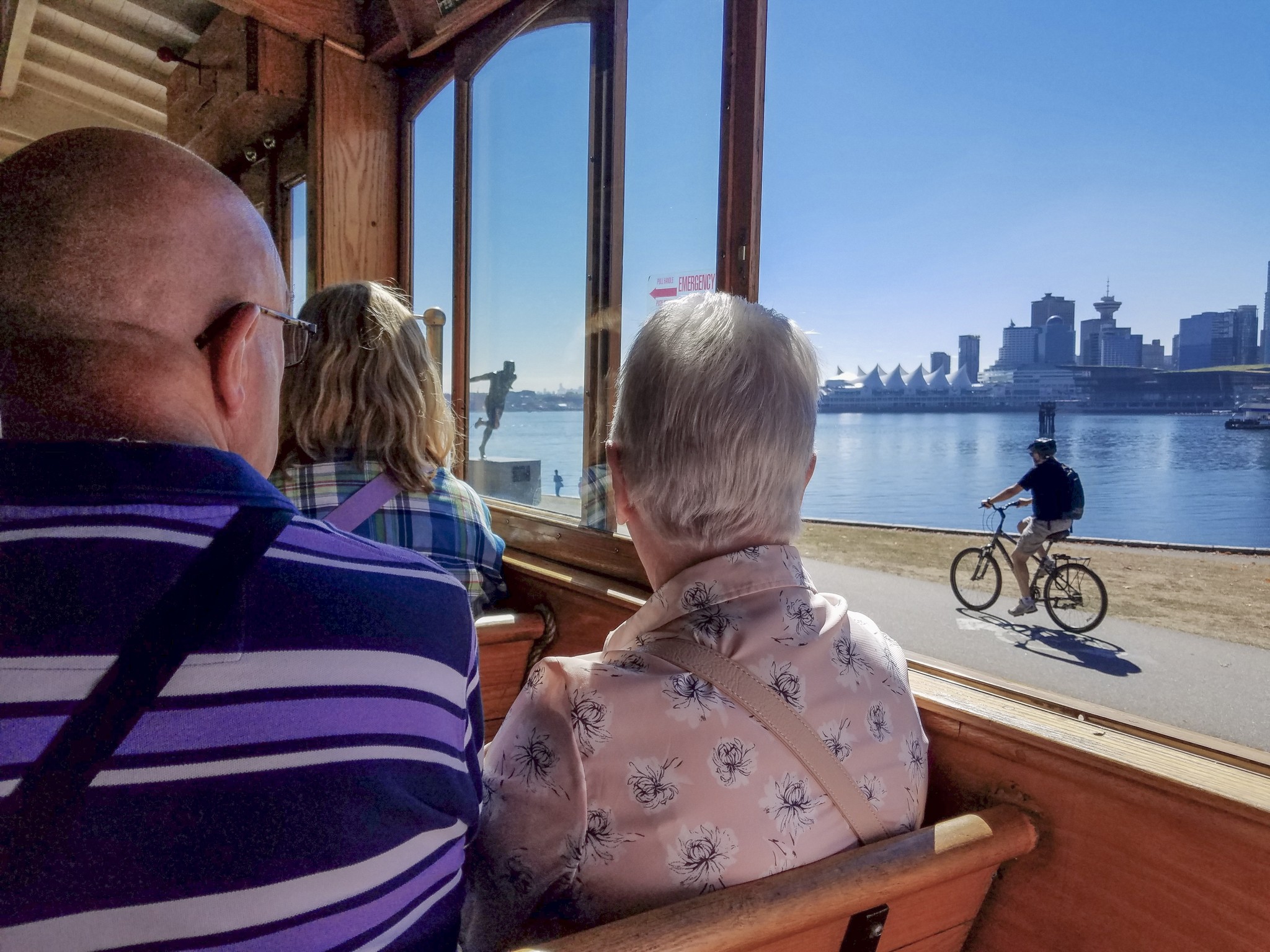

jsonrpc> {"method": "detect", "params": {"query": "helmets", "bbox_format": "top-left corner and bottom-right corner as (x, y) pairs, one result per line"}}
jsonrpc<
(1027, 436), (1056, 457)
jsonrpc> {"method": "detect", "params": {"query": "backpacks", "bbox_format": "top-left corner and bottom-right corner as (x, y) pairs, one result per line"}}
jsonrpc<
(1036, 462), (1085, 520)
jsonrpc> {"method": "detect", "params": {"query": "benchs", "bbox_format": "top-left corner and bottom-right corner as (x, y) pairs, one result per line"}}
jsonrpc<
(475, 603), (1043, 952)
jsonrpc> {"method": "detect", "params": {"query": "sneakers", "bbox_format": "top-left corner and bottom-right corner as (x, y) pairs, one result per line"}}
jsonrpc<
(1033, 561), (1055, 577)
(1008, 598), (1037, 616)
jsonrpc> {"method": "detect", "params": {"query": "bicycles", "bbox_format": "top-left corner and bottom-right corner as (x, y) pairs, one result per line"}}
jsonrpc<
(949, 500), (1108, 633)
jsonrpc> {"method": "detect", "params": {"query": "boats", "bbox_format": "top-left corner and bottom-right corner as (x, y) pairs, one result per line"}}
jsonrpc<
(1224, 401), (1270, 430)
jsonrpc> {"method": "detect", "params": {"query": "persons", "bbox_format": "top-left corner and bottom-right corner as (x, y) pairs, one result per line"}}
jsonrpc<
(469, 361), (517, 458)
(460, 291), (929, 952)
(578, 477), (582, 499)
(981, 437), (1073, 616)
(0, 126), (486, 952)
(554, 470), (563, 497)
(266, 282), (508, 617)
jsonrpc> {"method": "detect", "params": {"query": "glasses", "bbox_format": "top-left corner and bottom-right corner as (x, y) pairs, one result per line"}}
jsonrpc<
(1030, 451), (1035, 456)
(195, 301), (316, 368)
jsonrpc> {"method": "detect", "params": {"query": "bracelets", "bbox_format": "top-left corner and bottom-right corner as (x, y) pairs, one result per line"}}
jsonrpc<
(987, 498), (993, 505)
